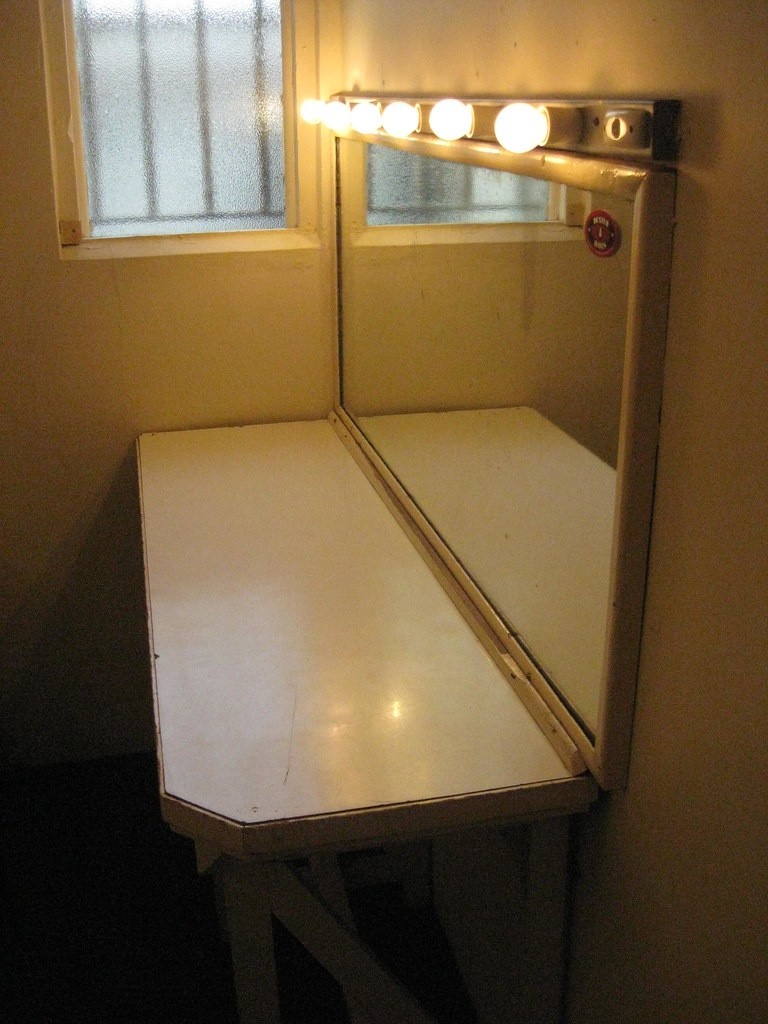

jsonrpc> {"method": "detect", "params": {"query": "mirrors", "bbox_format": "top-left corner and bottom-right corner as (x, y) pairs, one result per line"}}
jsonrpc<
(320, 121), (678, 793)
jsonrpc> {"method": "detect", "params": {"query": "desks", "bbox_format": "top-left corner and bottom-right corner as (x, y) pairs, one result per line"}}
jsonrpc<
(359, 407), (619, 741)
(138, 418), (592, 1024)
